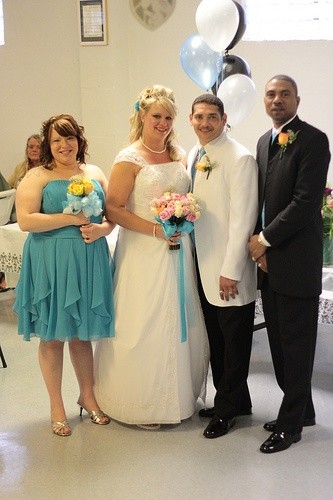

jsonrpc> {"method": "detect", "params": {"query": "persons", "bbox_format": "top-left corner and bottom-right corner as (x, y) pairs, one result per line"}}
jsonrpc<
(9, 135), (45, 190)
(92, 87), (211, 430)
(185, 94), (258, 439)
(246, 75), (332, 453)
(0, 174), (17, 293)
(15, 112), (117, 437)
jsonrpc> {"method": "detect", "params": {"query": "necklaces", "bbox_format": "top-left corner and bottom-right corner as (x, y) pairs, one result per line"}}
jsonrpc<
(139, 137), (166, 154)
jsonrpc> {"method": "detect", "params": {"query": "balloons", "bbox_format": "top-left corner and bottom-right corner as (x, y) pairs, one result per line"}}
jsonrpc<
(180, 34), (222, 89)
(217, 73), (256, 127)
(195, 0), (239, 52)
(212, 54), (252, 95)
(225, 0), (247, 50)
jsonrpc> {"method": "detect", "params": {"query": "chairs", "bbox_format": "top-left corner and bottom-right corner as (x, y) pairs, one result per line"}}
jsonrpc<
(0, 189), (17, 226)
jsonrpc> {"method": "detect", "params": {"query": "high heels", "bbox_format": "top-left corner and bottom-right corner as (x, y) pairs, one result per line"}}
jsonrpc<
(76, 402), (111, 425)
(51, 419), (72, 436)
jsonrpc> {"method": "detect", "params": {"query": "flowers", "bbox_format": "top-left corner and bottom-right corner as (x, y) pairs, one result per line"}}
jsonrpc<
(63, 175), (104, 217)
(277, 130), (295, 149)
(320, 188), (333, 241)
(195, 155), (216, 172)
(149, 190), (204, 251)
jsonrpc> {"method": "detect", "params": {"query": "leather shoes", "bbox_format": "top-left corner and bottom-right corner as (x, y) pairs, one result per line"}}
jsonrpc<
(199, 406), (252, 417)
(260, 431), (301, 453)
(263, 416), (315, 432)
(203, 414), (235, 439)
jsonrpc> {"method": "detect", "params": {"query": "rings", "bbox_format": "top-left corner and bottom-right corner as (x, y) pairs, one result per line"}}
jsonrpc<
(86, 235), (87, 238)
(220, 291), (223, 293)
(252, 257), (256, 261)
(229, 292), (232, 293)
(258, 264), (261, 266)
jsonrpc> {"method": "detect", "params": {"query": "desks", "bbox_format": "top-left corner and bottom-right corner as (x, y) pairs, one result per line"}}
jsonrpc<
(0, 221), (121, 288)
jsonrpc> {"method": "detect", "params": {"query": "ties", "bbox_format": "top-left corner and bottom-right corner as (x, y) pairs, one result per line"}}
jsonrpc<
(190, 147), (206, 247)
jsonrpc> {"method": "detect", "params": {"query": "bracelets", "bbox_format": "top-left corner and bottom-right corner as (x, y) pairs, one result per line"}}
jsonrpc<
(153, 226), (155, 238)
(258, 235), (265, 247)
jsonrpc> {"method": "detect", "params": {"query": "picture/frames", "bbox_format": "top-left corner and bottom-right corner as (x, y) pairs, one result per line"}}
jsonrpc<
(78, 0), (108, 46)
